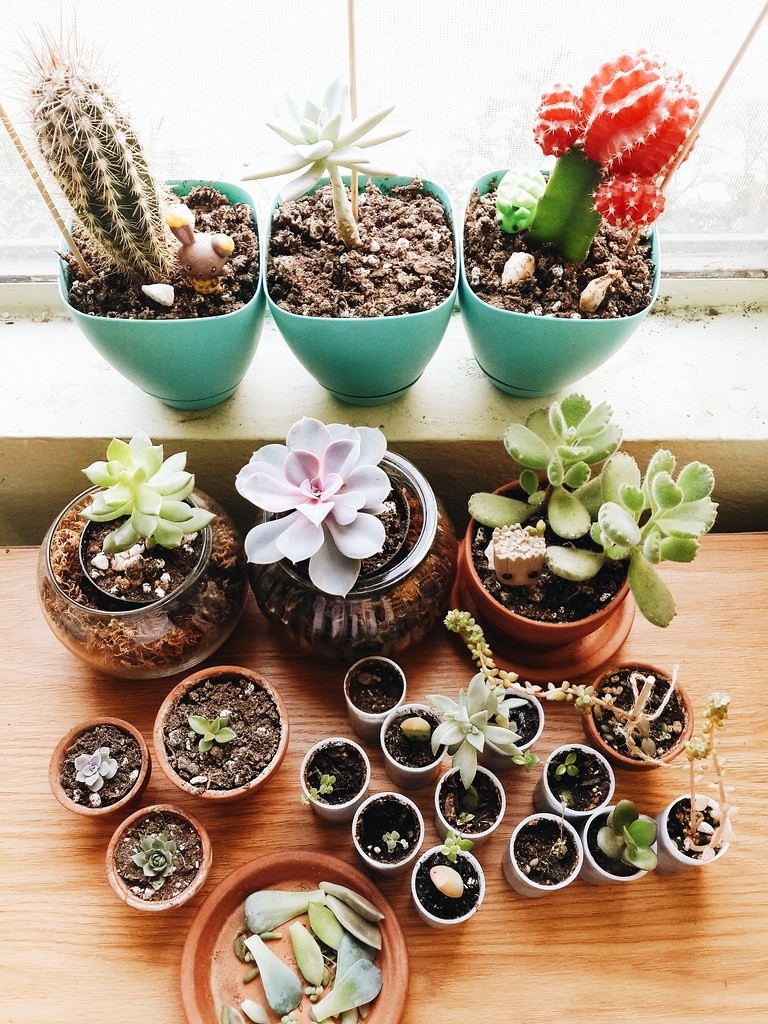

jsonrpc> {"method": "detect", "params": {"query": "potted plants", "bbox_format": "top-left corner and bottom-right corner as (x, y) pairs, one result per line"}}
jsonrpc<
(580, 798), (659, 886)
(48, 715), (152, 821)
(5, 6), (267, 411)
(444, 608), (694, 774)
(234, 415), (411, 599)
(299, 738), (371, 825)
(432, 766), (507, 851)
(104, 803), (214, 914)
(74, 428), (218, 612)
(449, 388), (720, 682)
(153, 664), (290, 805)
(380, 702), (450, 790)
(652, 690), (740, 877)
(239, 74), (460, 407)
(410, 829), (485, 930)
(351, 792), (425, 877)
(457, 46), (701, 400)
(501, 794), (585, 899)
(424, 669), (544, 791)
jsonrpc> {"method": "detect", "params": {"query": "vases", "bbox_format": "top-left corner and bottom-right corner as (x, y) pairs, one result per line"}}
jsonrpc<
(343, 656), (407, 742)
(249, 448), (459, 661)
(35, 483), (252, 680)
